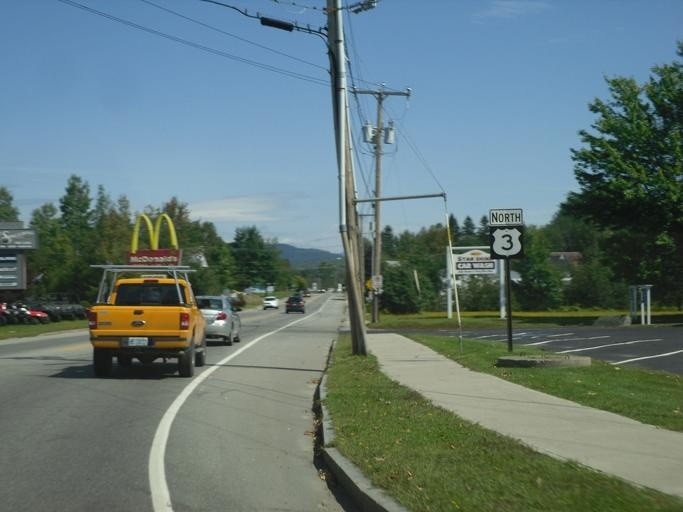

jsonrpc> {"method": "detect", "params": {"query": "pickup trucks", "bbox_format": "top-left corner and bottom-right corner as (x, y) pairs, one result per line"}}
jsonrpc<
(89, 277), (207, 377)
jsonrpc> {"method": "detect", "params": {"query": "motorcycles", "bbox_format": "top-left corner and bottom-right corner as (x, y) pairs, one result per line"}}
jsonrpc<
(0, 302), (86, 327)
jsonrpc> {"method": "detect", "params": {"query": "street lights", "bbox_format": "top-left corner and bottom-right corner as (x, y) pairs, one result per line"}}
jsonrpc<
(257, 15), (369, 356)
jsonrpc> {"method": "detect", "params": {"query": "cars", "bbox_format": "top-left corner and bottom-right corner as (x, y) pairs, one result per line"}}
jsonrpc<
(262, 296), (279, 311)
(195, 294), (242, 346)
(285, 295), (306, 314)
